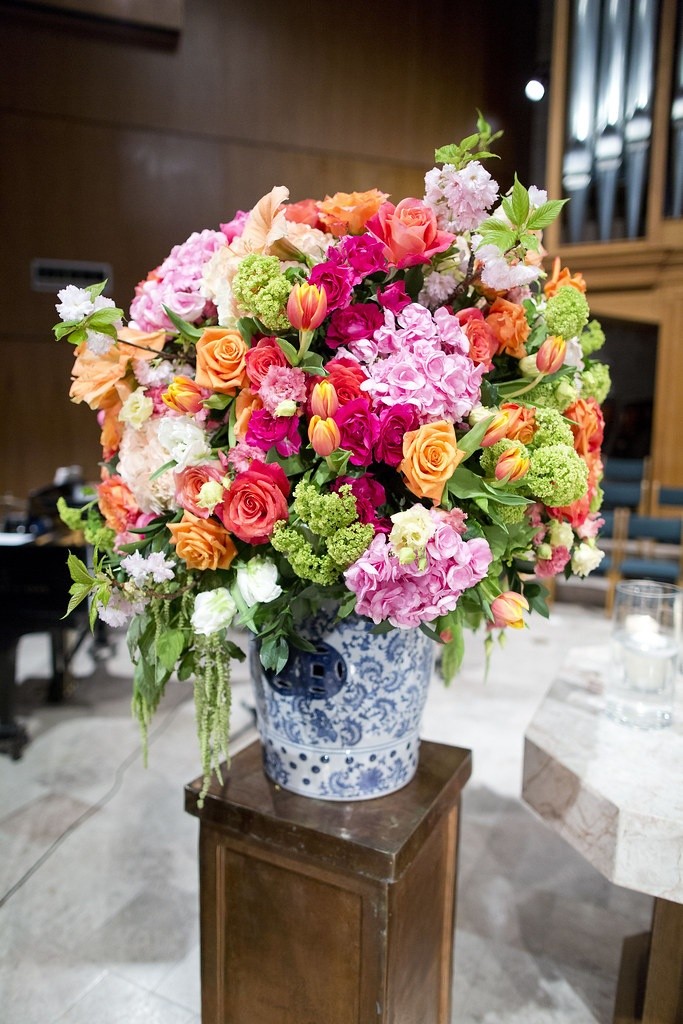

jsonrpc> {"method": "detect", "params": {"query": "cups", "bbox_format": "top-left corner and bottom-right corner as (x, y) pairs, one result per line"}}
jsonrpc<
(603, 578), (682, 729)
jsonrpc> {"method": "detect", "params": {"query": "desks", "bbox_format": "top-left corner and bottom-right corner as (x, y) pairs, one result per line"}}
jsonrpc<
(184, 739), (478, 1022)
(519, 636), (683, 1023)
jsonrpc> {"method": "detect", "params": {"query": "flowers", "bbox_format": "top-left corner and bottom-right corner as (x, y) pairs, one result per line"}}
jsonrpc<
(52, 111), (612, 806)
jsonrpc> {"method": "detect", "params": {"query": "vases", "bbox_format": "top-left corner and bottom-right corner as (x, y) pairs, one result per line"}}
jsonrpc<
(251, 619), (436, 802)
